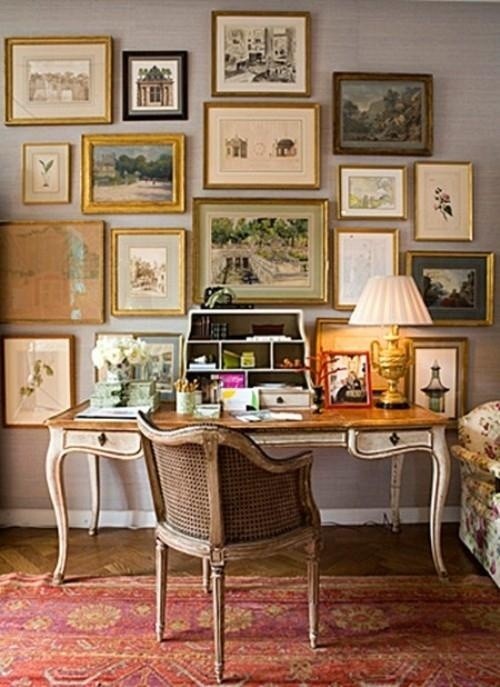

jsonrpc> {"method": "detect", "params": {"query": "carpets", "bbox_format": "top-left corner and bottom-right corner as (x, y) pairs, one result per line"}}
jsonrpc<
(0, 573), (500, 686)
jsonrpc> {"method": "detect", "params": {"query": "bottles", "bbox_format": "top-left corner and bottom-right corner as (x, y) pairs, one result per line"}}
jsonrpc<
(240, 351), (256, 368)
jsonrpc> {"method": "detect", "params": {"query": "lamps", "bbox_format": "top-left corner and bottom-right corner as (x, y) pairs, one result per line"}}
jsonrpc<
(348, 274), (433, 410)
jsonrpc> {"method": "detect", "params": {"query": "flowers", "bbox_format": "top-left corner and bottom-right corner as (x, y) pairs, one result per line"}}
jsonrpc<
(90, 333), (153, 370)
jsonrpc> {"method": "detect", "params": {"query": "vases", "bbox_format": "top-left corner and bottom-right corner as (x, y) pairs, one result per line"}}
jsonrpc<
(107, 367), (137, 382)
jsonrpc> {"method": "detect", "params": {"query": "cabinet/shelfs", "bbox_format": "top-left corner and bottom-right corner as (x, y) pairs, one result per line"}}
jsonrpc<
(184, 308), (318, 410)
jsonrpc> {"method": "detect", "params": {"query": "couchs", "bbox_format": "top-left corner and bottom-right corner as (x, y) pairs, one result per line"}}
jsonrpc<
(451, 400), (500, 588)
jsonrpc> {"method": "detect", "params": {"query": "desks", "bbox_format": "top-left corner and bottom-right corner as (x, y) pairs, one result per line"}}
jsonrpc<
(44, 400), (453, 587)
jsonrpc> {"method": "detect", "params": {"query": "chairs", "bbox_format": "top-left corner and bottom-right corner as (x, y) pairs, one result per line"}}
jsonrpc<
(134, 409), (323, 682)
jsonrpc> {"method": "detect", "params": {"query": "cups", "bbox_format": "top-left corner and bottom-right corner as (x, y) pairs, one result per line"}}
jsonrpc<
(175, 392), (195, 414)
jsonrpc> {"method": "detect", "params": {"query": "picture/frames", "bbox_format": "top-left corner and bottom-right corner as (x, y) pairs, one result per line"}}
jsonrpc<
(4, 35), (113, 124)
(315, 317), (403, 391)
(410, 336), (468, 430)
(122, 50), (187, 120)
